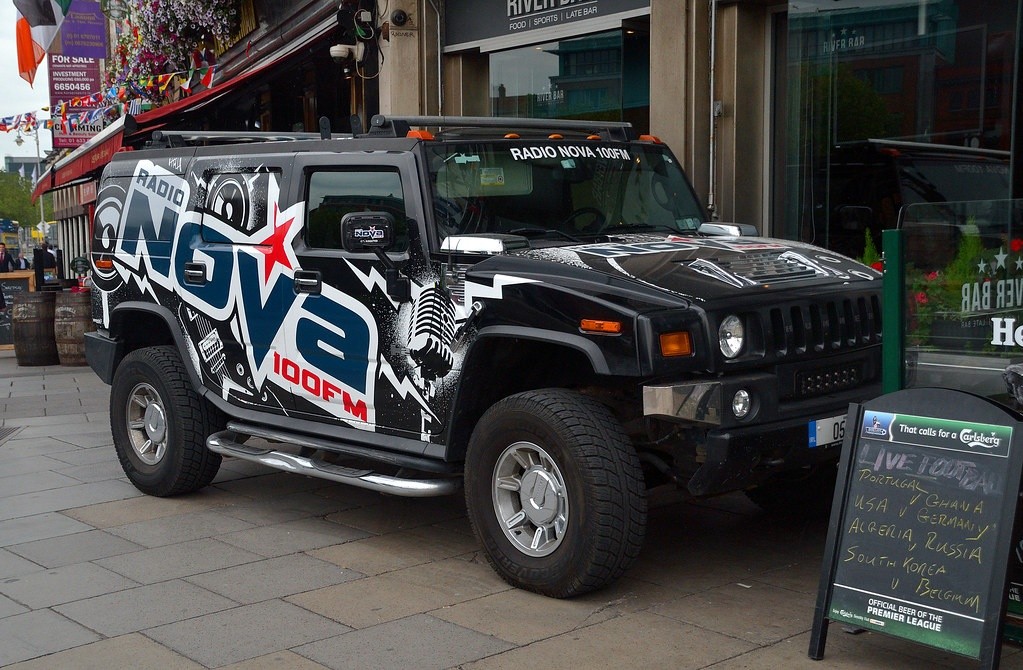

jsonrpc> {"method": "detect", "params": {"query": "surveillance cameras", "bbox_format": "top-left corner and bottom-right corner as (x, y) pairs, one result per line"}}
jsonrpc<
(329, 40), (365, 64)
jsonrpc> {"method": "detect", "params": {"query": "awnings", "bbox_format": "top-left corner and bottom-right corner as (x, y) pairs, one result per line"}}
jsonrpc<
(33, 24), (335, 205)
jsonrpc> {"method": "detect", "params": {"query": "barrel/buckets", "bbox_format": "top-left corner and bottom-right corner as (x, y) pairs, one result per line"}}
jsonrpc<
(54, 293), (96, 366)
(11, 291), (60, 366)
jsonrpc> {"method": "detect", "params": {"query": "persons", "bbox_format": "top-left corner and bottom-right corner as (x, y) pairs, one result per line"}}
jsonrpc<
(15, 253), (30, 270)
(32, 244), (57, 269)
(0, 243), (17, 273)
(0, 286), (9, 311)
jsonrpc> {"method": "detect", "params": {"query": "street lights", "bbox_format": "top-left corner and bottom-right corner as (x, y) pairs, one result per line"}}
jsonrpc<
(14, 120), (45, 237)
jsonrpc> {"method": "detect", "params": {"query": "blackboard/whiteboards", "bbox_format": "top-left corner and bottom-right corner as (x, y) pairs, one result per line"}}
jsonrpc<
(0, 273), (35, 350)
(825, 401), (1023, 658)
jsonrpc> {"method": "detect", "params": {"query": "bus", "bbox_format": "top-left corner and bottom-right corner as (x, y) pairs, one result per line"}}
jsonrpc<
(30, 220), (58, 245)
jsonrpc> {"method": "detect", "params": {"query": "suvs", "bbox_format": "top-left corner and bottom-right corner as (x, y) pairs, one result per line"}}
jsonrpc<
(831, 136), (1011, 274)
(83, 115), (919, 598)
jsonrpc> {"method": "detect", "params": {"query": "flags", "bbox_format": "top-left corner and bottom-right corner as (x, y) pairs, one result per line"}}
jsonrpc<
(12, 0), (71, 86)
(0, 66), (217, 134)
